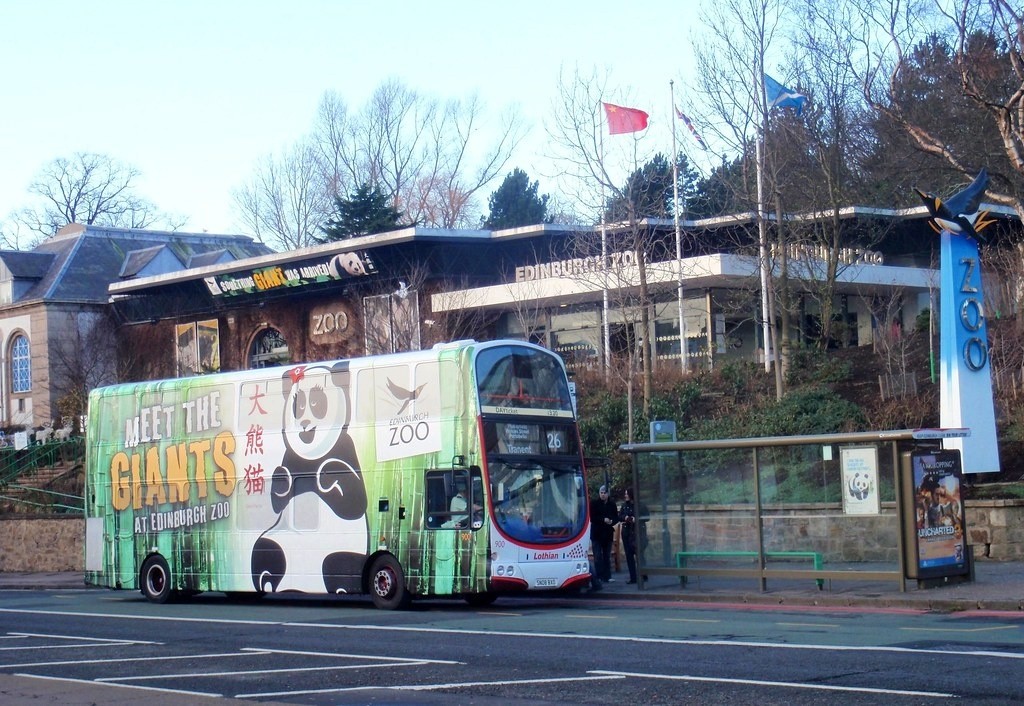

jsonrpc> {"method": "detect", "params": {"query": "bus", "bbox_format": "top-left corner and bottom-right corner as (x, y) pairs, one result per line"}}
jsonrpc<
(84, 339), (612, 609)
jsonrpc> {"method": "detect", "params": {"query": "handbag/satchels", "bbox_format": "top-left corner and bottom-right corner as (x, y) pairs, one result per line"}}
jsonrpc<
(630, 523), (649, 554)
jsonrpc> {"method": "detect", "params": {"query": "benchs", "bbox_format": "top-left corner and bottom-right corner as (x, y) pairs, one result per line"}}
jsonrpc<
(675, 550), (823, 589)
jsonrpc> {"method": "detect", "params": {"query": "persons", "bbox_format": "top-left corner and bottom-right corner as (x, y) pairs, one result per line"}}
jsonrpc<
(588, 566), (604, 593)
(590, 485), (619, 583)
(619, 487), (650, 584)
(450, 485), (484, 526)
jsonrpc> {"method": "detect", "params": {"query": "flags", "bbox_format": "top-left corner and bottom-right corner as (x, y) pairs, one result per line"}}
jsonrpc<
(676, 106), (707, 149)
(764, 73), (806, 117)
(603, 103), (649, 135)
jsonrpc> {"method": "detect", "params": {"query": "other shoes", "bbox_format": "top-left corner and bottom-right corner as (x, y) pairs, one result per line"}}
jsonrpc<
(626, 577), (637, 584)
(642, 575), (648, 581)
(608, 578), (616, 583)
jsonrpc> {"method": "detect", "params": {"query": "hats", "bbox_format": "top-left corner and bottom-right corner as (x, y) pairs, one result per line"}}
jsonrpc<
(600, 485), (610, 496)
